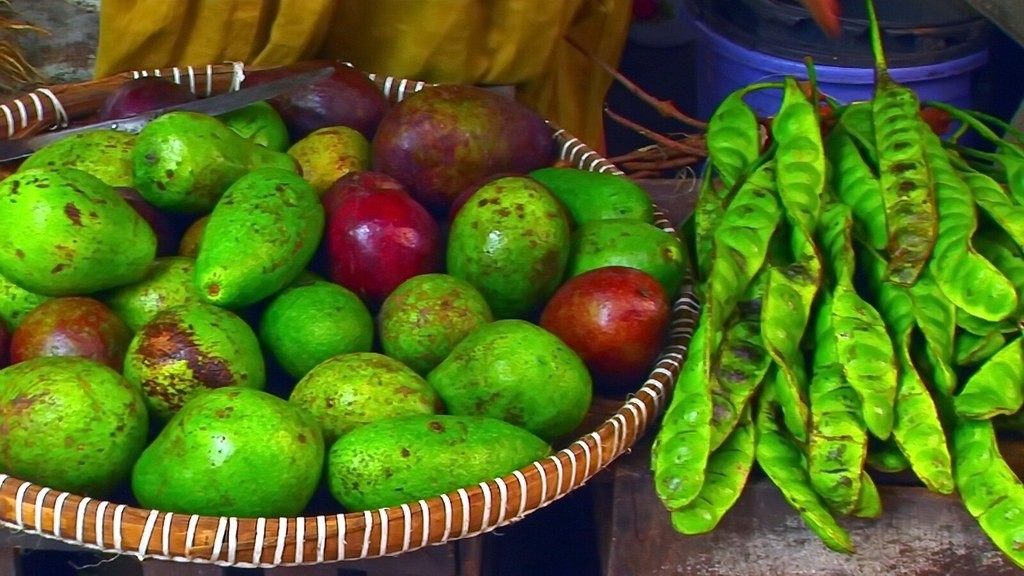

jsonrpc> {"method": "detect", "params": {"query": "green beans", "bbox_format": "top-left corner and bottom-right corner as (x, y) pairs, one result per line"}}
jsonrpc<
(651, 0), (1024, 574)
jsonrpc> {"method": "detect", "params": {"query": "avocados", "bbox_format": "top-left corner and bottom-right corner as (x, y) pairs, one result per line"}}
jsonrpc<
(0, 59), (683, 511)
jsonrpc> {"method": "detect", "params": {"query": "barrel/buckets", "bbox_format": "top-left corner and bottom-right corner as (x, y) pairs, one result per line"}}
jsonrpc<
(684, 0), (993, 181)
